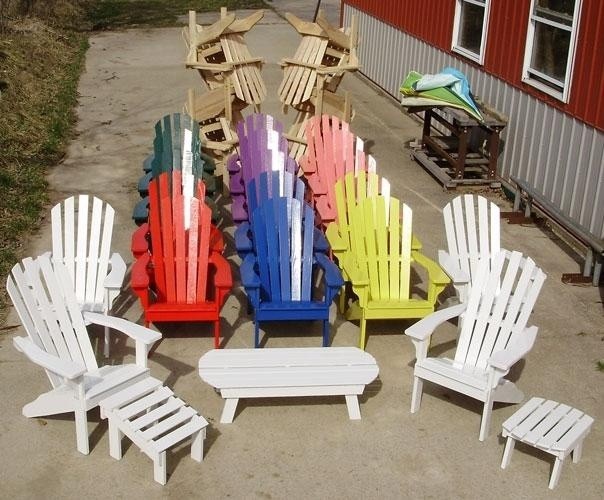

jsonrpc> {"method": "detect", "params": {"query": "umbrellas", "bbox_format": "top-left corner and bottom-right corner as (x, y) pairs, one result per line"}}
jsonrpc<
(398, 66), (491, 128)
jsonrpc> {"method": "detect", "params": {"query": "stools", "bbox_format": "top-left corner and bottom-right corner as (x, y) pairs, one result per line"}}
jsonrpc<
(501, 397), (594, 490)
(99, 377), (209, 486)
